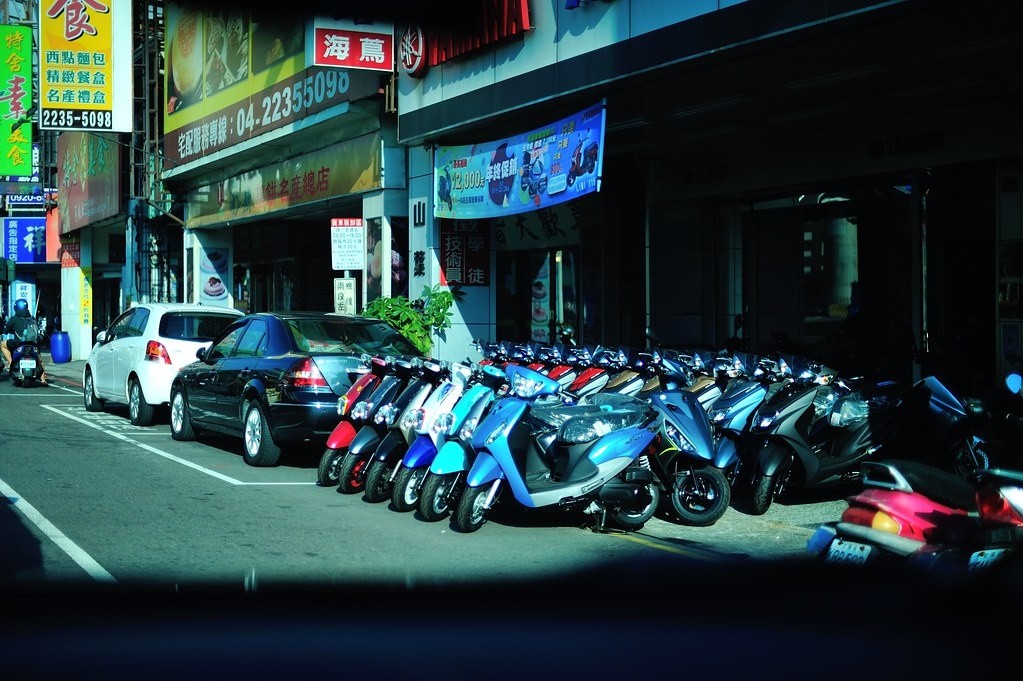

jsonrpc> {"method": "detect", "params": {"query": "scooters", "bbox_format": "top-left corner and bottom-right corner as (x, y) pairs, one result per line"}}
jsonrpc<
(315, 337), (990, 533)
(2, 325), (44, 389)
(806, 456), (1023, 621)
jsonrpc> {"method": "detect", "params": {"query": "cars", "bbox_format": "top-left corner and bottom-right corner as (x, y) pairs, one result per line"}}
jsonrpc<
(168, 310), (427, 468)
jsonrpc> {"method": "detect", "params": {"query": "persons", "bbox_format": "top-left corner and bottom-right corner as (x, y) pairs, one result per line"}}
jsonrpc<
(0, 299), (48, 384)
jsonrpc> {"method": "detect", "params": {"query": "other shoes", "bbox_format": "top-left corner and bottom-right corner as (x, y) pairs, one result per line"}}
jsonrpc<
(3, 363), (11, 371)
(40, 379), (48, 386)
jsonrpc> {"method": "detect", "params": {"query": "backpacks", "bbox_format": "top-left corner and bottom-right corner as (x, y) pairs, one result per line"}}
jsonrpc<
(13, 315), (37, 344)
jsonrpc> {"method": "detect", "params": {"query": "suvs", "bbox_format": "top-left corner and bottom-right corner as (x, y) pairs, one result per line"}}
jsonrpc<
(81, 300), (250, 426)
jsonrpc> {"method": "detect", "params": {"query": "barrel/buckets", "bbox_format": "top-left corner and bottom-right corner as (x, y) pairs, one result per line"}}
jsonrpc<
(50, 331), (71, 363)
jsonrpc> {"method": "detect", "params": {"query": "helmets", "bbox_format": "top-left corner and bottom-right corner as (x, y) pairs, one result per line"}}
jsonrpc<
(14, 299), (29, 313)
